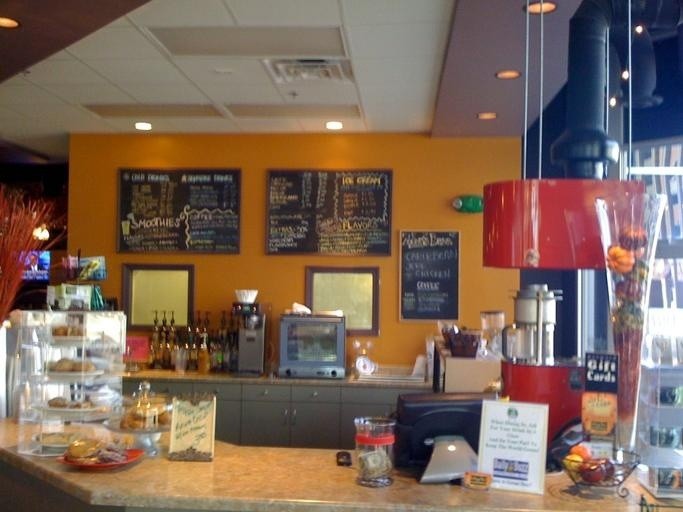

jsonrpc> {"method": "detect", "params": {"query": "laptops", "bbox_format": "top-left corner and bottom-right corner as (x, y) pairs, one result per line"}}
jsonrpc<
(66, 437), (104, 464)
(119, 394), (170, 429)
(47, 328), (94, 408)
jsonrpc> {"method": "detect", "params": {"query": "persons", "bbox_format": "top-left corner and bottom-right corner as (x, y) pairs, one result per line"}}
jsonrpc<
(23, 254), (45, 270)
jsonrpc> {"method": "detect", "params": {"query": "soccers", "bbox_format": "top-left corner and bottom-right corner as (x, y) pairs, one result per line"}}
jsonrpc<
(562, 455), (583, 480)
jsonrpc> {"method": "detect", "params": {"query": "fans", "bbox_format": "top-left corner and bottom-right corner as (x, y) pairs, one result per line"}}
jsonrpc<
(264, 167), (393, 258)
(167, 391), (218, 462)
(398, 229), (461, 323)
(116, 166), (241, 255)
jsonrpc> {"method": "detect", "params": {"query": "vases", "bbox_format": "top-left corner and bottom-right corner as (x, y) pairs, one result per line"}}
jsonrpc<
(595, 196), (668, 454)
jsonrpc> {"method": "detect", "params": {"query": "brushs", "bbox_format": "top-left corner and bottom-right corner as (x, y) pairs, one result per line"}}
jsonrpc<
(501, 283), (564, 367)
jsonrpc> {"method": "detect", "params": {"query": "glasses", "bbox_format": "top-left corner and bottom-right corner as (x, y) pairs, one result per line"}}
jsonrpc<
(482, 0), (646, 271)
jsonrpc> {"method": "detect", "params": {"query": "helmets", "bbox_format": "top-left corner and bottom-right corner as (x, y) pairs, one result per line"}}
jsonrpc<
(355, 356), (377, 376)
(55, 448), (145, 470)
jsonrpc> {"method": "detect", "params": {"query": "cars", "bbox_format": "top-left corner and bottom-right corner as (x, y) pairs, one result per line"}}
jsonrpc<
(353, 417), (397, 487)
(649, 385), (683, 493)
(594, 194), (670, 487)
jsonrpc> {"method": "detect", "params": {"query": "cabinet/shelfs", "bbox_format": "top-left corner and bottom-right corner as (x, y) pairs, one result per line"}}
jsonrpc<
(149, 309), (237, 373)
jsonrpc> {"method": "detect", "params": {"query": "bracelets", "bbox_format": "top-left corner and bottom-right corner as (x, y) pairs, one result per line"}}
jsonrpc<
(235, 289), (258, 304)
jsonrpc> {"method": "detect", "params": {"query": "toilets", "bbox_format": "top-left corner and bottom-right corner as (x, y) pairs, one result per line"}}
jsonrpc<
(545, 415), (589, 473)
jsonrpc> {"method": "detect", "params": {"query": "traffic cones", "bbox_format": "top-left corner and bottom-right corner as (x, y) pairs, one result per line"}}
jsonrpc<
(606, 227), (648, 303)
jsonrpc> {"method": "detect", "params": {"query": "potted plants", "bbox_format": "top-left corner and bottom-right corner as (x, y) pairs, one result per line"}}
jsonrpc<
(122, 263), (194, 333)
(305, 269), (379, 338)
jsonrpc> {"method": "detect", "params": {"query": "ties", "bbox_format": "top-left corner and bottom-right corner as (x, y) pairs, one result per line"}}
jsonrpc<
(12, 248), (54, 282)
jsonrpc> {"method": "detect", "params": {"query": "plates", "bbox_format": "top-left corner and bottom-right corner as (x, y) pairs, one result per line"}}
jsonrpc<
(20, 335), (126, 453)
(123, 379), (395, 447)
(636, 364), (683, 500)
